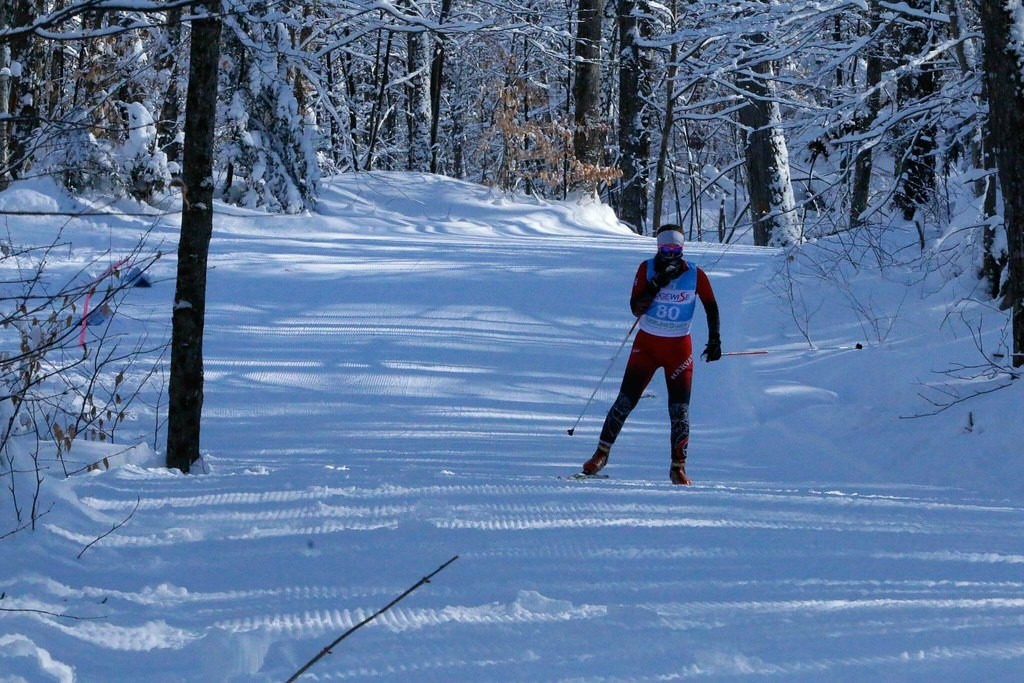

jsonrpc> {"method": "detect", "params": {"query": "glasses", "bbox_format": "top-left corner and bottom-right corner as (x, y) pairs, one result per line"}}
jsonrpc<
(659, 244), (683, 256)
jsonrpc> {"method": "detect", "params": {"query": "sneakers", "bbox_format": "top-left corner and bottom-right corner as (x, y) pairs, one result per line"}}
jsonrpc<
(583, 447), (608, 474)
(670, 467), (690, 485)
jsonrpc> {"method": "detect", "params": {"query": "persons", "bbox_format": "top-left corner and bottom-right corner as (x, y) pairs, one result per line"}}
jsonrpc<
(582, 224), (721, 485)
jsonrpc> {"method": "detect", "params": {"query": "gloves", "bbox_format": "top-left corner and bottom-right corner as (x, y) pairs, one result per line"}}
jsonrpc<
(650, 264), (676, 293)
(700, 340), (721, 362)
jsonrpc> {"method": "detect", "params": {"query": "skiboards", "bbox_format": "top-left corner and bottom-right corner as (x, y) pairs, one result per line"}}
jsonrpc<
(555, 470), (610, 481)
